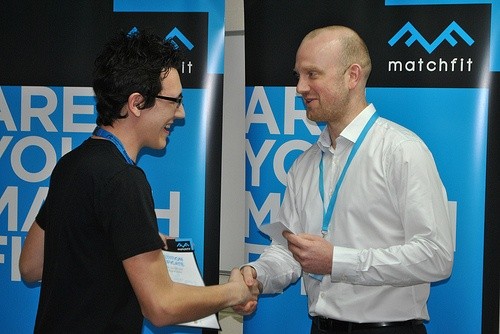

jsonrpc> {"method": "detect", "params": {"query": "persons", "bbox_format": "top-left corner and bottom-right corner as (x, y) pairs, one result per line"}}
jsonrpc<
(231, 26), (455, 334)
(18, 31), (260, 334)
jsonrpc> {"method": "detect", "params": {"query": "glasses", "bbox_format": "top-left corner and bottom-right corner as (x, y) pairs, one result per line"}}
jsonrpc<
(155, 93), (184, 108)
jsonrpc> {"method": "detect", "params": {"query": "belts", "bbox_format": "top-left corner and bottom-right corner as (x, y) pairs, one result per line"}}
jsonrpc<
(312, 315), (422, 333)
